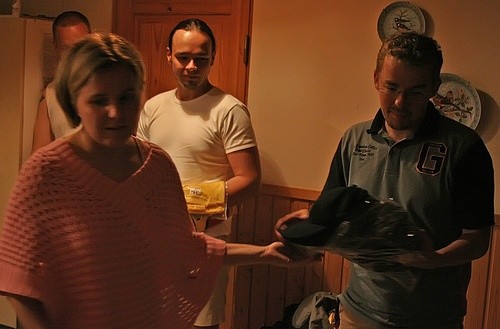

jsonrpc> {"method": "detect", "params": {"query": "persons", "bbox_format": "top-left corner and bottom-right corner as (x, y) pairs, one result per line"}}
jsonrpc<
(135, 17), (262, 329)
(29, 11), (92, 157)
(274, 27), (495, 329)
(0, 28), (325, 329)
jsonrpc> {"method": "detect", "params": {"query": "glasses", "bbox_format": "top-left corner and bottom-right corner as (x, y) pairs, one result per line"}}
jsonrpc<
(377, 76), (430, 102)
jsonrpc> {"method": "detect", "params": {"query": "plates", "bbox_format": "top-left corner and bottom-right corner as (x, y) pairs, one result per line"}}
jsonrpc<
(429, 73), (482, 130)
(278, 223), (408, 256)
(377, 1), (426, 43)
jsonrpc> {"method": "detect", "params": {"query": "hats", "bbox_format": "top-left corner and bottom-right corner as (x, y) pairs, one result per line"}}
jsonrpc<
(280, 183), (372, 242)
